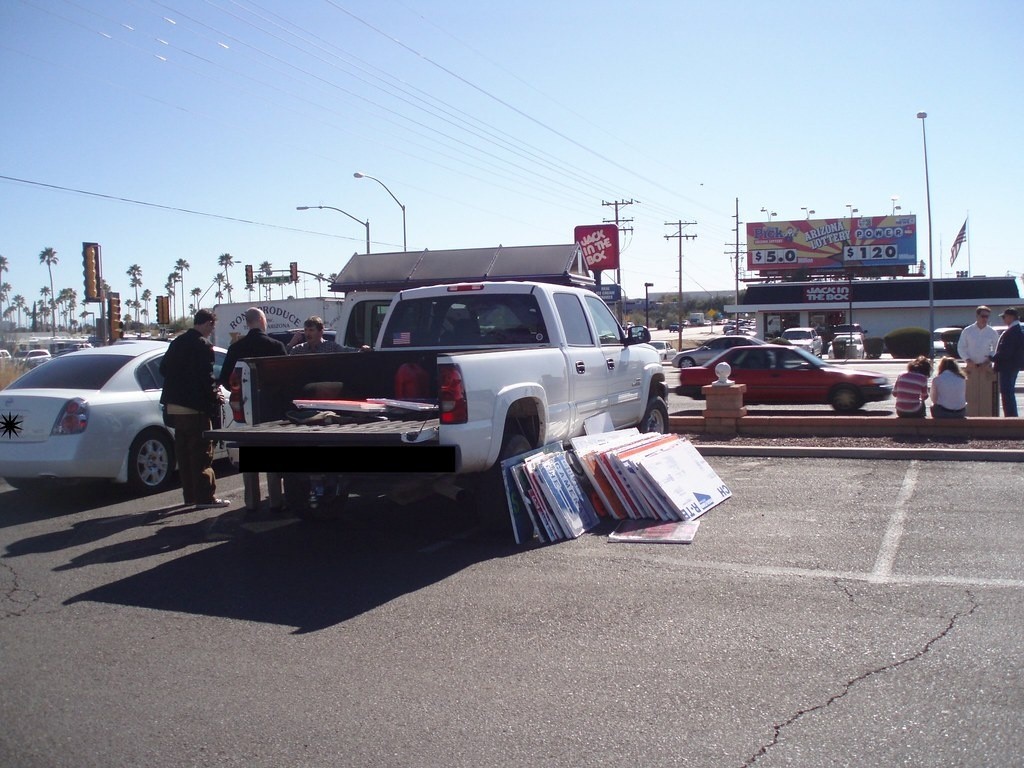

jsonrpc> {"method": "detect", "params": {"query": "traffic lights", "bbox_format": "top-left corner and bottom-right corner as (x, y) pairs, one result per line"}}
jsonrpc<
(290, 262), (297, 281)
(81, 241), (100, 302)
(245, 264), (253, 284)
(107, 292), (124, 341)
(155, 295), (163, 324)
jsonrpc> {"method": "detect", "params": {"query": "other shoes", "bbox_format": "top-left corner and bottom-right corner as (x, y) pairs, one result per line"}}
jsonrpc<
(185, 499), (199, 506)
(196, 499), (230, 509)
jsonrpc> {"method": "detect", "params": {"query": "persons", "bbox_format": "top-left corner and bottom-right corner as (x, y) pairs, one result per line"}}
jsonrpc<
(285, 315), (373, 355)
(218, 306), (287, 511)
(930, 357), (968, 418)
(956, 306), (999, 417)
(160, 308), (230, 508)
(892, 355), (933, 418)
(988, 307), (1024, 417)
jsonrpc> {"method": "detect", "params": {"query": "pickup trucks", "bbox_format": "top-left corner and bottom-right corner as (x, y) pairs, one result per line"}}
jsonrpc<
(202, 282), (670, 530)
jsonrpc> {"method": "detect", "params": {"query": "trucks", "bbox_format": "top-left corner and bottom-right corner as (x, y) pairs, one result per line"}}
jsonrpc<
(690, 312), (704, 326)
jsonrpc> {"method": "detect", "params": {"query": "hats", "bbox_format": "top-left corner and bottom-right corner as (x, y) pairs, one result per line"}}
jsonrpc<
(998, 308), (1017, 317)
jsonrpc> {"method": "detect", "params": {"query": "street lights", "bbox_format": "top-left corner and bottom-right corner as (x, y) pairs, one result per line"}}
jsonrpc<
(917, 112), (935, 364)
(644, 282), (654, 330)
(354, 173), (407, 252)
(297, 205), (371, 253)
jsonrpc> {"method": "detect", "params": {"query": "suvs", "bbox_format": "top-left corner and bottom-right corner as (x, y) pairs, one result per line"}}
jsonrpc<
(775, 321), (869, 360)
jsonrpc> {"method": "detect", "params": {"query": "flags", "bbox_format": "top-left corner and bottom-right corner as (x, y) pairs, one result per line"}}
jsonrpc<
(950, 220), (967, 267)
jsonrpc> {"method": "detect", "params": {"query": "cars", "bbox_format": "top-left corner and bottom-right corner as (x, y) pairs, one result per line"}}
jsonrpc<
(0, 328), (339, 495)
(704, 318), (756, 336)
(933, 323), (1024, 351)
(677, 344), (894, 415)
(648, 341), (677, 361)
(672, 335), (775, 368)
(668, 319), (691, 333)
(622, 321), (635, 330)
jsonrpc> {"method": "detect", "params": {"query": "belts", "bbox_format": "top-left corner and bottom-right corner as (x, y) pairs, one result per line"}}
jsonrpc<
(938, 405), (964, 412)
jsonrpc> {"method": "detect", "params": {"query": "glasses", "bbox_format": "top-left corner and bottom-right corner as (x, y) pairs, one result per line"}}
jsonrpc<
(979, 314), (988, 319)
